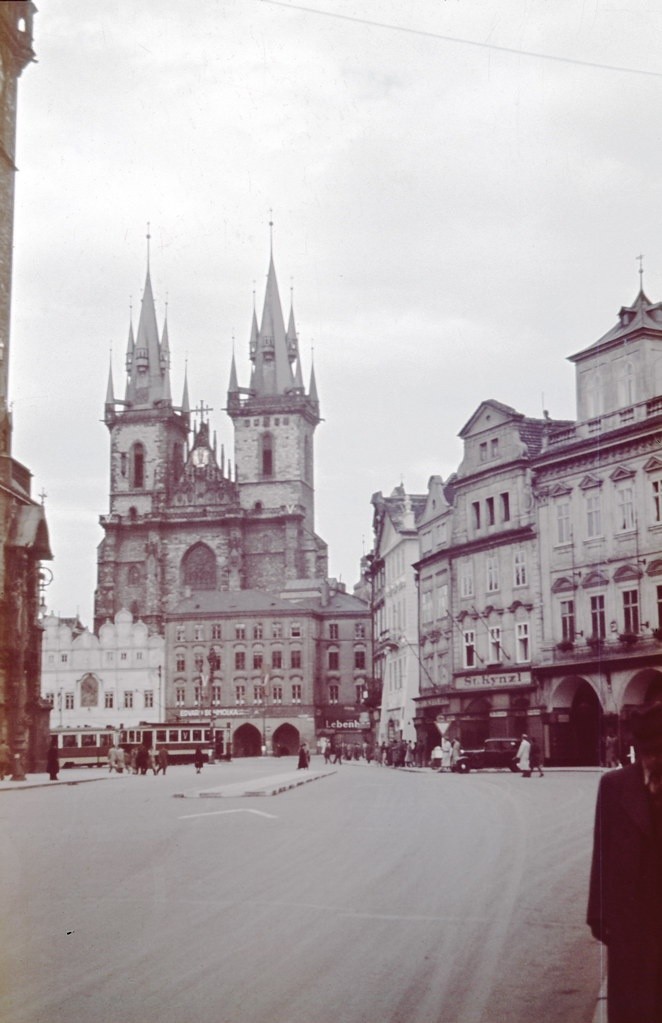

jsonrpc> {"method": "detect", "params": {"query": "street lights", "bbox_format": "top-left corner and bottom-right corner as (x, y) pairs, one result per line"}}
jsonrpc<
(207, 646), (217, 764)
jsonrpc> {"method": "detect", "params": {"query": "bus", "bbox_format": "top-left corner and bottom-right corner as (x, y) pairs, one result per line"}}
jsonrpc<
(48, 727), (120, 768)
(120, 721), (213, 765)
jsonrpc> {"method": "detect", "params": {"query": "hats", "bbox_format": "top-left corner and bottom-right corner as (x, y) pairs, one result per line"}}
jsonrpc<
(627, 702), (662, 747)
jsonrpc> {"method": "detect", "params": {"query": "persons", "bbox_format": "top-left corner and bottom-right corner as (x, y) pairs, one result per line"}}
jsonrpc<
(586, 705), (662, 1023)
(439, 737), (460, 772)
(606, 733), (618, 768)
(195, 746), (202, 774)
(297, 743), (310, 770)
(513, 734), (544, 777)
(108, 744), (168, 776)
(0, 740), (12, 781)
(46, 742), (59, 780)
(366, 739), (429, 768)
(324, 741), (361, 764)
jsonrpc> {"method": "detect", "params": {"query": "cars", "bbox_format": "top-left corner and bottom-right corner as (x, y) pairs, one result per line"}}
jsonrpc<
(450, 738), (521, 772)
